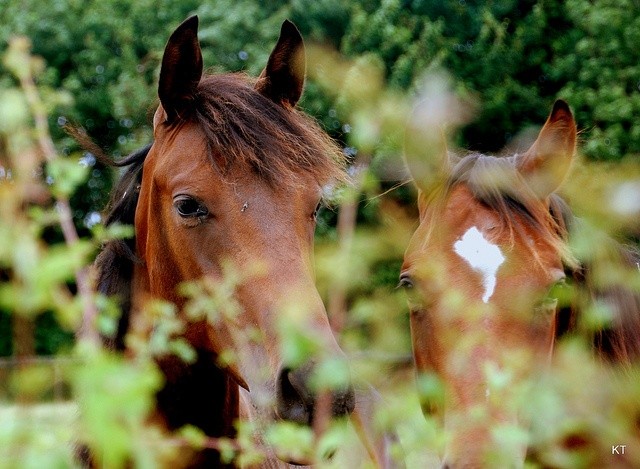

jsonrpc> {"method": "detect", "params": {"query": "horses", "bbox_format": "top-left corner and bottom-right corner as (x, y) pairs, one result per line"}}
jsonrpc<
(57, 15), (355, 469)
(391, 100), (640, 469)
